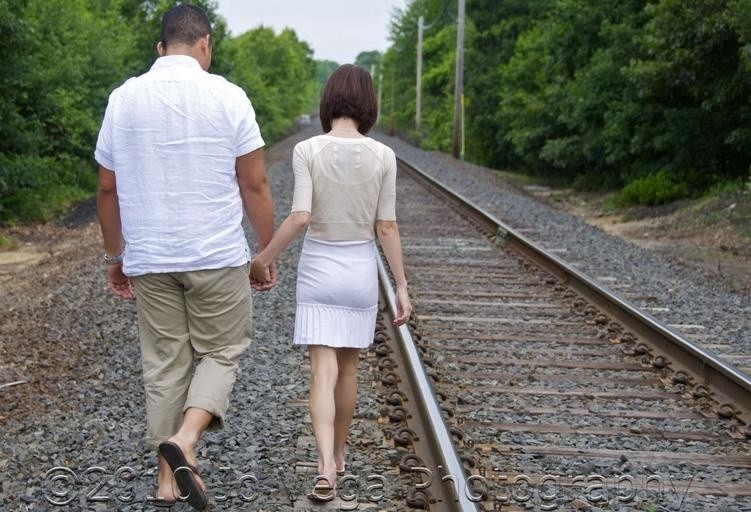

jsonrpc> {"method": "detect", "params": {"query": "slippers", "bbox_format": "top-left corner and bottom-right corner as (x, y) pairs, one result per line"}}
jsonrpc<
(159, 440), (211, 511)
(155, 493), (177, 508)
(336, 461), (345, 476)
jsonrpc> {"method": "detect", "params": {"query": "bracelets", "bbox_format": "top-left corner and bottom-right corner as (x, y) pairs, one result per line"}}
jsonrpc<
(103, 249), (126, 266)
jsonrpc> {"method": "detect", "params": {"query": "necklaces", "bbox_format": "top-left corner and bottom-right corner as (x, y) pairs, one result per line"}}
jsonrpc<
(333, 128), (358, 132)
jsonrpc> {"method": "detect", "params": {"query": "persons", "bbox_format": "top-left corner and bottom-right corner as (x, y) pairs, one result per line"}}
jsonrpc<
(93, 6), (276, 508)
(249, 63), (417, 504)
